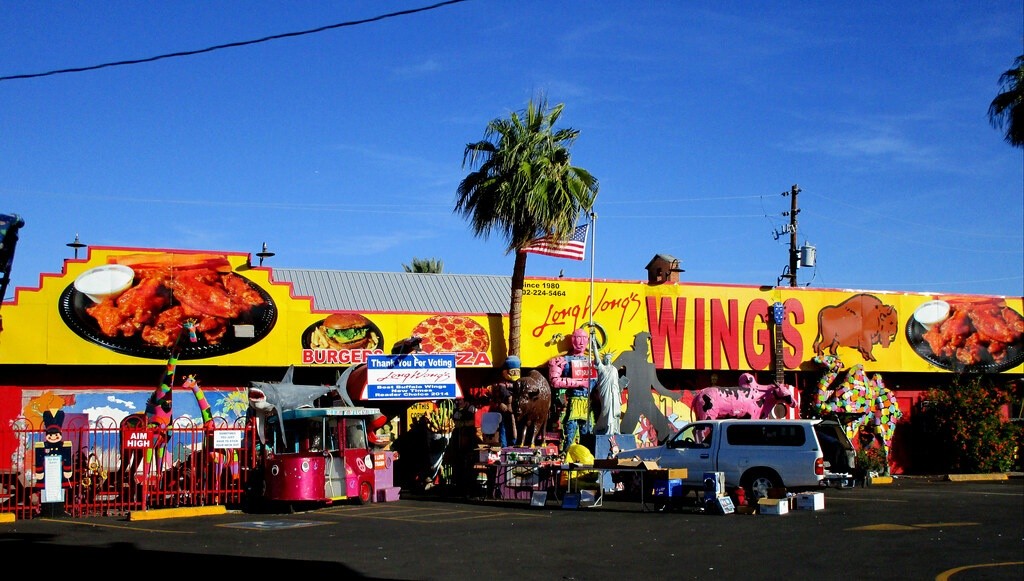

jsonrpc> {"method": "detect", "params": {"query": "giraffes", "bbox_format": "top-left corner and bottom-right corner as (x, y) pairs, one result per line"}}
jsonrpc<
(181, 374), (240, 503)
(114, 317), (201, 505)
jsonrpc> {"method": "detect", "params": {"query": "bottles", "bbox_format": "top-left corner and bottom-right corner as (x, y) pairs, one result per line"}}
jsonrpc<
(328, 432), (335, 450)
(305, 437), (310, 450)
(295, 440), (299, 454)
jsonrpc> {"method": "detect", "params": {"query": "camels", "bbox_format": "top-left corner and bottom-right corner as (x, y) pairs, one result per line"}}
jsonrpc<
(809, 355), (905, 475)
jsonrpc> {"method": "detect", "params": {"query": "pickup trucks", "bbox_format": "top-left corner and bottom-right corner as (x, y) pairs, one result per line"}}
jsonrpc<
(614, 419), (858, 500)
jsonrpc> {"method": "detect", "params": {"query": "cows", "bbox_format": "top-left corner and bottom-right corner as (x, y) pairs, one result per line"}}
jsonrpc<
(690, 378), (798, 449)
(505, 369), (552, 448)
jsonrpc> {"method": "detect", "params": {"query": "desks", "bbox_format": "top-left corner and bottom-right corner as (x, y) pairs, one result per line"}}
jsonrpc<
(484, 464), (652, 513)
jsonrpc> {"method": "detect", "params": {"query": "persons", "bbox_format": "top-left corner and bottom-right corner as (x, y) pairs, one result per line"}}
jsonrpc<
(588, 329), (622, 435)
(548, 329), (599, 452)
(492, 356), (523, 447)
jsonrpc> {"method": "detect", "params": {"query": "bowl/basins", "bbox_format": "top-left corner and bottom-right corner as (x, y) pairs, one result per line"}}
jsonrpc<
(913, 300), (950, 331)
(74, 264), (136, 303)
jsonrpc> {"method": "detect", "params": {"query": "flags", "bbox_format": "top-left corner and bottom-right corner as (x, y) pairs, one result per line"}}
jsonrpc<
(520, 224), (589, 260)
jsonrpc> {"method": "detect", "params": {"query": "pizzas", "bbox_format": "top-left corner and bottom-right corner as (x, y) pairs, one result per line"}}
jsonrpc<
(413, 314), (489, 357)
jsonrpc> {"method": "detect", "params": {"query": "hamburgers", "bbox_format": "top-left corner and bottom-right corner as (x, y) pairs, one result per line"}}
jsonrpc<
(323, 314), (369, 350)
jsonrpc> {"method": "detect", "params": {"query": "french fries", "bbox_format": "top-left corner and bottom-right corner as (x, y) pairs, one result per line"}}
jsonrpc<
(311, 327), (329, 348)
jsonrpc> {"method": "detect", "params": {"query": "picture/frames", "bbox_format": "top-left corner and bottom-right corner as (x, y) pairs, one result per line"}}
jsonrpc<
(561, 493), (581, 509)
(530, 490), (547, 507)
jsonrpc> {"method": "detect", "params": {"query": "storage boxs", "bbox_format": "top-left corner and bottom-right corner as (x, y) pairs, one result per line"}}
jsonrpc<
(593, 457), (688, 500)
(757, 497), (789, 515)
(797, 491), (824, 510)
